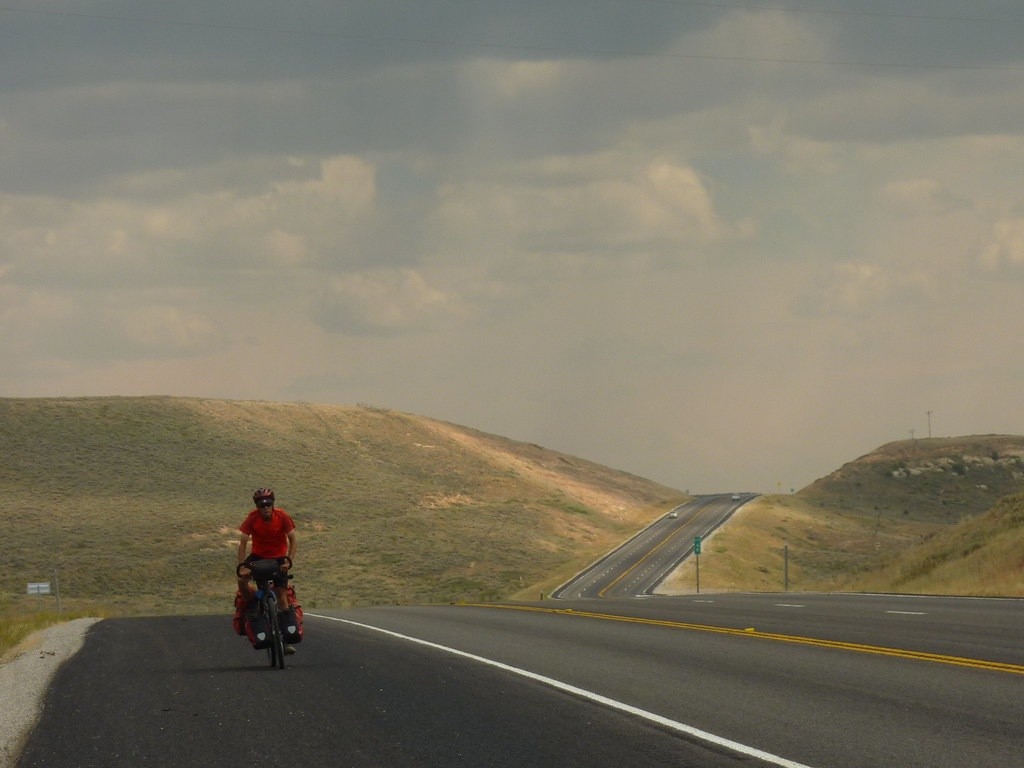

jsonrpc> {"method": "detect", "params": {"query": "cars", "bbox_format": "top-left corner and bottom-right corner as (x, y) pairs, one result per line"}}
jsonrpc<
(668, 511), (678, 520)
(731, 494), (739, 501)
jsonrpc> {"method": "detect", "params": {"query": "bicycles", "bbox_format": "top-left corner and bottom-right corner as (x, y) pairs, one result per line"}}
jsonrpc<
(237, 556), (296, 669)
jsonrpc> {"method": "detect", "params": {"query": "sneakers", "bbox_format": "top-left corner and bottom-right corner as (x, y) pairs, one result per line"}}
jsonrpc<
(284, 646), (297, 656)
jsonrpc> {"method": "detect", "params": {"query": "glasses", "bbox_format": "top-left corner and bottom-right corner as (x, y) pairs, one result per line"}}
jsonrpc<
(256, 501), (273, 508)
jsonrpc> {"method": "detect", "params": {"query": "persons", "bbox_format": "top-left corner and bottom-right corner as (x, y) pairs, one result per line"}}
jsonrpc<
(239, 488), (297, 656)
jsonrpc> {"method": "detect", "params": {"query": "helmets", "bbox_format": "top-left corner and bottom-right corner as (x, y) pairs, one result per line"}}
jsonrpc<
(253, 488), (275, 505)
